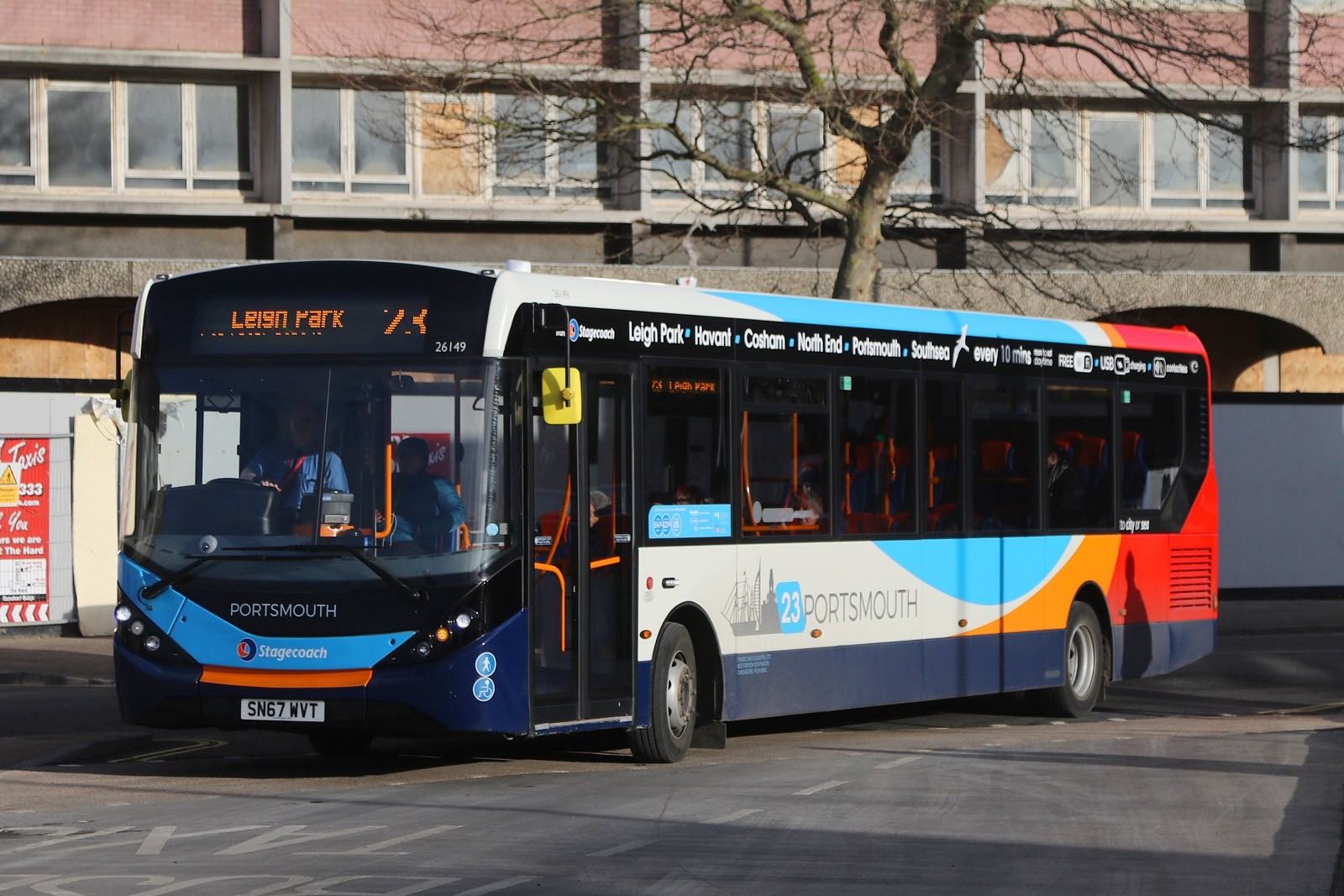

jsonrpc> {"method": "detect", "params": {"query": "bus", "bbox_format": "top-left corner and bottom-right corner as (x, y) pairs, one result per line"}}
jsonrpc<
(109, 257), (1221, 764)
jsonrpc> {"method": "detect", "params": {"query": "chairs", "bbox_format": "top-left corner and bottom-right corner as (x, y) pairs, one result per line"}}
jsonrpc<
(533, 429), (1152, 577)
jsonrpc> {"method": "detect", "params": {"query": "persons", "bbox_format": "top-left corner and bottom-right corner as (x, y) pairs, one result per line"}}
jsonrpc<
(372, 436), (467, 557)
(239, 395), (350, 535)
(589, 388), (1085, 580)
